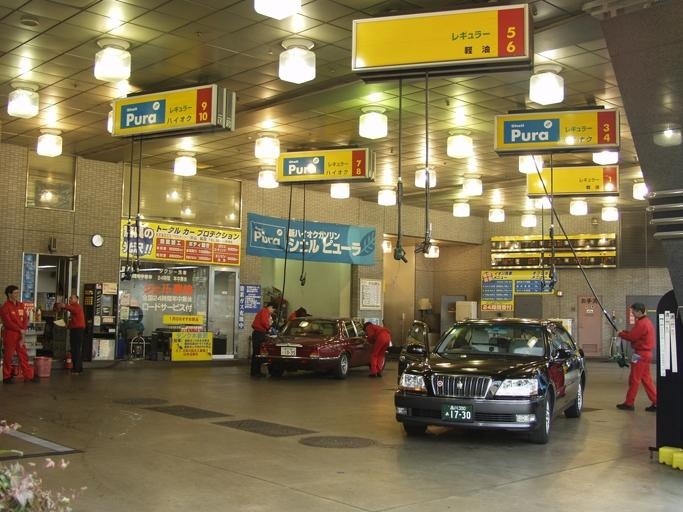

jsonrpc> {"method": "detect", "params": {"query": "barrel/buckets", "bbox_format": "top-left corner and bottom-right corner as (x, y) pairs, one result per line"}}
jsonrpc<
(149, 350), (157, 361)
(157, 351), (163, 361)
(35, 356), (52, 377)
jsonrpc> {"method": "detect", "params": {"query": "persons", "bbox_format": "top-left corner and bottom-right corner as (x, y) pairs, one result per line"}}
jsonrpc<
(59, 294), (85, 374)
(251, 301), (278, 377)
(288, 307), (307, 321)
(363, 321), (392, 377)
(1, 285), (41, 384)
(616, 302), (657, 412)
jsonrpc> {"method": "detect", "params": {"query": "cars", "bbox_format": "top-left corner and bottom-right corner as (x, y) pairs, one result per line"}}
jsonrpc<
(258, 318), (389, 375)
(393, 317), (589, 445)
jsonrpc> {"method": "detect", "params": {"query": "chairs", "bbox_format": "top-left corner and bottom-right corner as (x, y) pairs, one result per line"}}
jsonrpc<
(511, 339), (545, 355)
(320, 327), (336, 336)
(290, 326), (305, 336)
(488, 337), (513, 353)
(463, 330), (499, 353)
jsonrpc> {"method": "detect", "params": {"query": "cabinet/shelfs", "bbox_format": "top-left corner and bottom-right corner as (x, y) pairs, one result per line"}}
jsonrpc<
(9, 321), (46, 375)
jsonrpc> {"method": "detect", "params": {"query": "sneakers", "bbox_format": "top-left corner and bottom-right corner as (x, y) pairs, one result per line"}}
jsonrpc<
(645, 404), (658, 411)
(615, 403), (635, 411)
(2, 378), (16, 384)
(24, 378), (41, 384)
